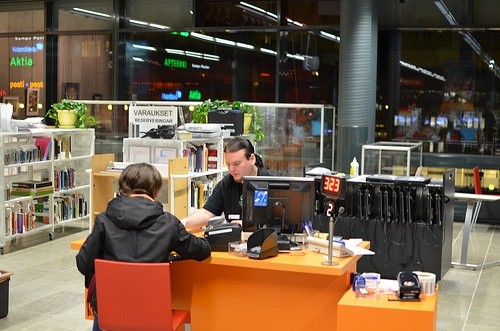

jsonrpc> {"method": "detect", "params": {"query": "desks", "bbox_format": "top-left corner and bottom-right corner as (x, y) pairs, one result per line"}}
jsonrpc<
(336, 279), (439, 331)
(71, 228), (370, 331)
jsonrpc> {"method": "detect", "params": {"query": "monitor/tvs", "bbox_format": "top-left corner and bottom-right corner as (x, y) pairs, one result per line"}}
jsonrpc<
(242, 176), (315, 253)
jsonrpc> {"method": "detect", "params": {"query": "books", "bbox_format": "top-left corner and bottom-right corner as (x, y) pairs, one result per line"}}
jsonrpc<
(53, 134), (72, 160)
(5, 201), (35, 235)
(4, 137), (49, 165)
(191, 179), (217, 210)
(54, 167), (76, 192)
(182, 142), (209, 172)
(207, 148), (226, 169)
(35, 193), (87, 224)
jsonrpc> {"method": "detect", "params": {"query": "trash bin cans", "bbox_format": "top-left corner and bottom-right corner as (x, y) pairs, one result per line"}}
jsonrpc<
(0, 269), (13, 319)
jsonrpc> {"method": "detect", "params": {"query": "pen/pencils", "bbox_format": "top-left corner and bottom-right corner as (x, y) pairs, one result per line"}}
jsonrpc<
(301, 220), (313, 236)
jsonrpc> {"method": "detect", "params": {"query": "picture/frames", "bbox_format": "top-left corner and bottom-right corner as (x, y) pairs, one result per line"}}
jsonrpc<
(3, 96), (19, 116)
(26, 88), (40, 116)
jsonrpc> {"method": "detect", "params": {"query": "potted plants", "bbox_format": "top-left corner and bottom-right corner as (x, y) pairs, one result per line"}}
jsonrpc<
(45, 99), (96, 129)
(193, 98), (264, 144)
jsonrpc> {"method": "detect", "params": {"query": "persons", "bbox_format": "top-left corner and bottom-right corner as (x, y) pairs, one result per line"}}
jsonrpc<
(76, 163), (211, 331)
(180, 136), (282, 230)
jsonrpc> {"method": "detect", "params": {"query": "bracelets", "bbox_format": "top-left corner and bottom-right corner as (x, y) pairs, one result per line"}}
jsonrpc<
(181, 219), (188, 226)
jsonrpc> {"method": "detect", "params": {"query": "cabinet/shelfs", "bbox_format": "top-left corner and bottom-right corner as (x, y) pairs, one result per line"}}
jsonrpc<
(92, 135), (254, 229)
(0, 129), (95, 253)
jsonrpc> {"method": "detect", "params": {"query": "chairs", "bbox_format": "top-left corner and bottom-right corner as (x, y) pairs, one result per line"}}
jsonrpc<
(95, 259), (191, 331)
(471, 166), (500, 232)
